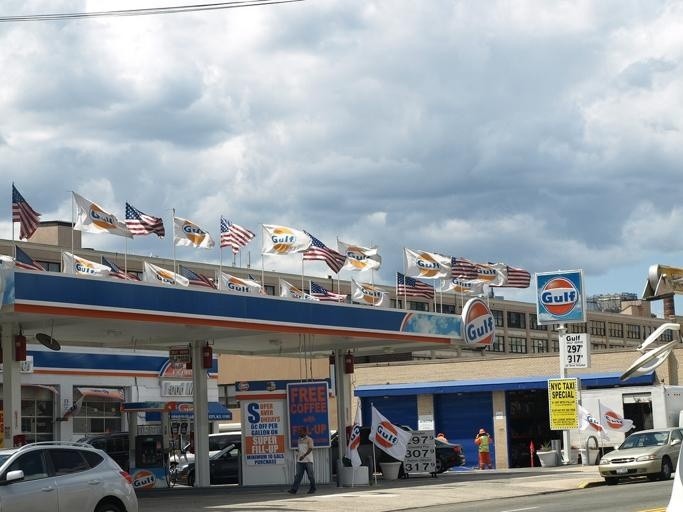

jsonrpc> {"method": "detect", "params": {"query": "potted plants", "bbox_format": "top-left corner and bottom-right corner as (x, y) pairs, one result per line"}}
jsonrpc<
(561, 445), (581, 465)
(378, 449), (402, 480)
(536, 441), (557, 467)
(579, 442), (600, 465)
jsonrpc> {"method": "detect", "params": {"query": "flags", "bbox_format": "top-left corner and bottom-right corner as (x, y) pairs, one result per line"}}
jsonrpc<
(219, 217), (261, 296)
(72, 192), (165, 238)
(336, 240), (389, 307)
(344, 406), (363, 471)
(62, 252), (218, 290)
(600, 403), (636, 433)
(173, 217), (214, 249)
(262, 224), (347, 302)
(368, 406), (413, 462)
(14, 244), (45, 271)
(396, 249), (531, 299)
(576, 403), (610, 441)
(11, 186), (41, 239)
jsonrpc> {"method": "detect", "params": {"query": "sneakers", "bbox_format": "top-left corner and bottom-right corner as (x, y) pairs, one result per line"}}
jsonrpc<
(287, 489), (296, 494)
(307, 488), (317, 493)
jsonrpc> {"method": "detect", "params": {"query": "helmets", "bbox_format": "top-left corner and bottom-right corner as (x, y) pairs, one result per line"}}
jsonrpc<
(437, 434), (444, 437)
(478, 429), (485, 435)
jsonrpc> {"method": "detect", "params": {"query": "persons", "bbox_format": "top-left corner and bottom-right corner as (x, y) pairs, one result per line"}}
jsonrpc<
(434, 433), (453, 474)
(288, 426), (317, 494)
(474, 428), (493, 469)
(641, 404), (653, 430)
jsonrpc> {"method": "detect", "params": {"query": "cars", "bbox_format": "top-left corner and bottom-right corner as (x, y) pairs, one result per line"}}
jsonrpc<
(77, 423), (241, 487)
(599, 427), (683, 486)
(330, 426), (465, 480)
(0, 441), (139, 512)
